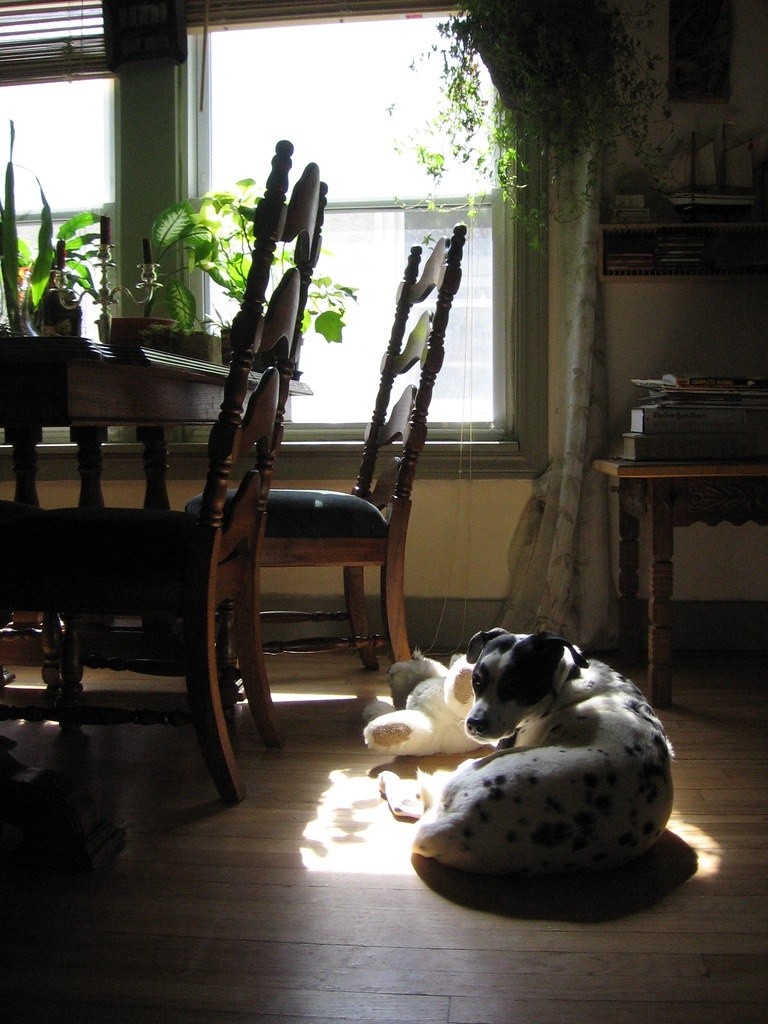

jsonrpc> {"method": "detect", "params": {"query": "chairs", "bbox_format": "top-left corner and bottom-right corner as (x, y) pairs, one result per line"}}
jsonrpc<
(174, 220), (469, 730)
(0, 138), (328, 808)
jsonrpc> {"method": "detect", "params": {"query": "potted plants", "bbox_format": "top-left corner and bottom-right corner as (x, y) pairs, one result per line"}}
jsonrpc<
(0, 120), (359, 381)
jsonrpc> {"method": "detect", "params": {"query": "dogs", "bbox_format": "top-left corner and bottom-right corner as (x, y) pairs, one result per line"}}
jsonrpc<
(378, 624), (676, 874)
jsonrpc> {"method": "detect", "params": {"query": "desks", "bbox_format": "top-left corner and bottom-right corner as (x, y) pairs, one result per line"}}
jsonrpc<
(592, 456), (768, 706)
(0, 331), (315, 788)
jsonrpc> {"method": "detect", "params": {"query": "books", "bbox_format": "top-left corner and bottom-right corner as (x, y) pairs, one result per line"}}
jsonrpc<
(621, 370), (768, 462)
(603, 232), (712, 271)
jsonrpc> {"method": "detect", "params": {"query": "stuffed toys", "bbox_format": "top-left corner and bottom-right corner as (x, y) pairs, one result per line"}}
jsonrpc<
(362, 645), (486, 755)
